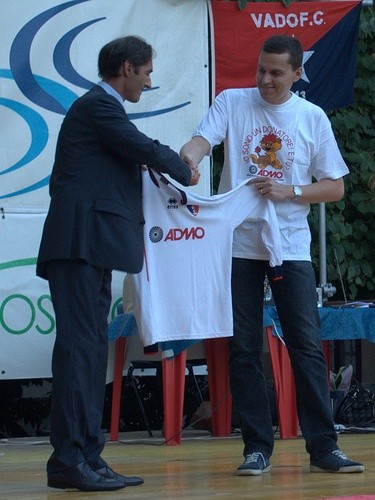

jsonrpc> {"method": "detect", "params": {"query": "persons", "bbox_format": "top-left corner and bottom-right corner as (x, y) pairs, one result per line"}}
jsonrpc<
(180, 35), (364, 475)
(36, 36), (200, 490)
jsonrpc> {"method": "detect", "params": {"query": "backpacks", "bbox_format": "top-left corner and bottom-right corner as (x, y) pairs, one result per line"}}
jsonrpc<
(330, 363), (354, 422)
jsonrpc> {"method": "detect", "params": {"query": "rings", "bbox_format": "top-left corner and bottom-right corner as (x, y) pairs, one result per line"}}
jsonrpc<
(260, 189), (263, 194)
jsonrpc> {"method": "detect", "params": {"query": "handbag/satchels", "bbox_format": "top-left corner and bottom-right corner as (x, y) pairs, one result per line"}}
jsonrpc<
(334, 384), (375, 433)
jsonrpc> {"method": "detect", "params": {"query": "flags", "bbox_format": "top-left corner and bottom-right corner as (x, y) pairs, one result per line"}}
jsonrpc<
(207, 0), (363, 110)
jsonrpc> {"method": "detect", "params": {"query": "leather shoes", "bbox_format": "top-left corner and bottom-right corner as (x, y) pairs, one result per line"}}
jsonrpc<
(95, 464), (144, 487)
(46, 463), (127, 492)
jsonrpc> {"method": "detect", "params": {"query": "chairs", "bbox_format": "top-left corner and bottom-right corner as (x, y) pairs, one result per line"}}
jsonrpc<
(124, 359), (214, 438)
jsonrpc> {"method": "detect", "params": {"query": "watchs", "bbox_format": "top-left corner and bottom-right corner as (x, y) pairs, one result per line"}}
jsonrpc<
(292, 185), (302, 201)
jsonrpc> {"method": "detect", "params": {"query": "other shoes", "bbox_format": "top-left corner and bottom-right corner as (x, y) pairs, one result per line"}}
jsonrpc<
(190, 412), (214, 434)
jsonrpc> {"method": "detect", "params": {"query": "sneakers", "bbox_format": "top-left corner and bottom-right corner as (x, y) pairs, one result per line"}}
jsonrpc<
(234, 450), (272, 477)
(310, 449), (364, 473)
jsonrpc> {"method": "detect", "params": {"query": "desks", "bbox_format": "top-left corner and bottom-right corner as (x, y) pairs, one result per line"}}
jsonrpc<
(105, 307), (375, 448)
